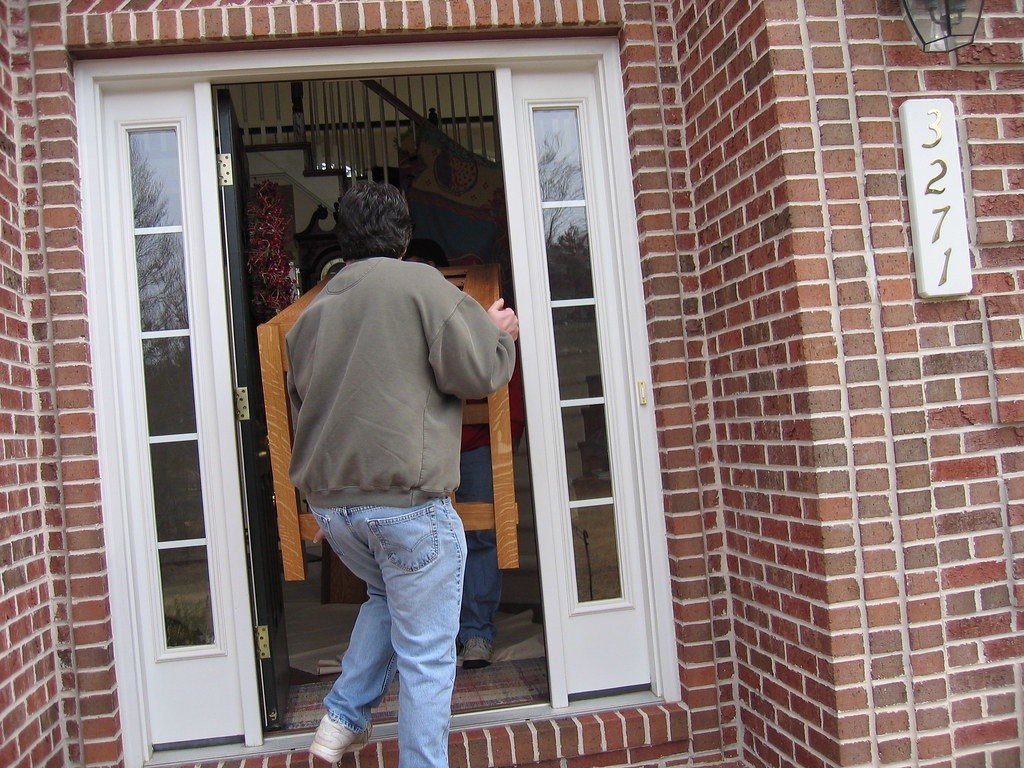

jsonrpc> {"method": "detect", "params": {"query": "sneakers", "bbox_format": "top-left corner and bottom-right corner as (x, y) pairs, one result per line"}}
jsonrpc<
(462, 637), (492, 669)
(308, 712), (373, 763)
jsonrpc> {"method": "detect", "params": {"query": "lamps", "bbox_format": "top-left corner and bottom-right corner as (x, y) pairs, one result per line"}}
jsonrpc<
(898, 0), (984, 53)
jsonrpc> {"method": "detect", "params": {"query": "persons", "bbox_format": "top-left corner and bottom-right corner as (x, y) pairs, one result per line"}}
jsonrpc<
(287, 177), (527, 768)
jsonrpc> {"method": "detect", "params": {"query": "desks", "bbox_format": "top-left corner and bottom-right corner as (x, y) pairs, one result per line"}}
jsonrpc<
(255, 262), (520, 581)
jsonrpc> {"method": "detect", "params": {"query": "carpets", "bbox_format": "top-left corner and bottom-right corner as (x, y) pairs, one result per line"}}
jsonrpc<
(282, 654), (548, 732)
